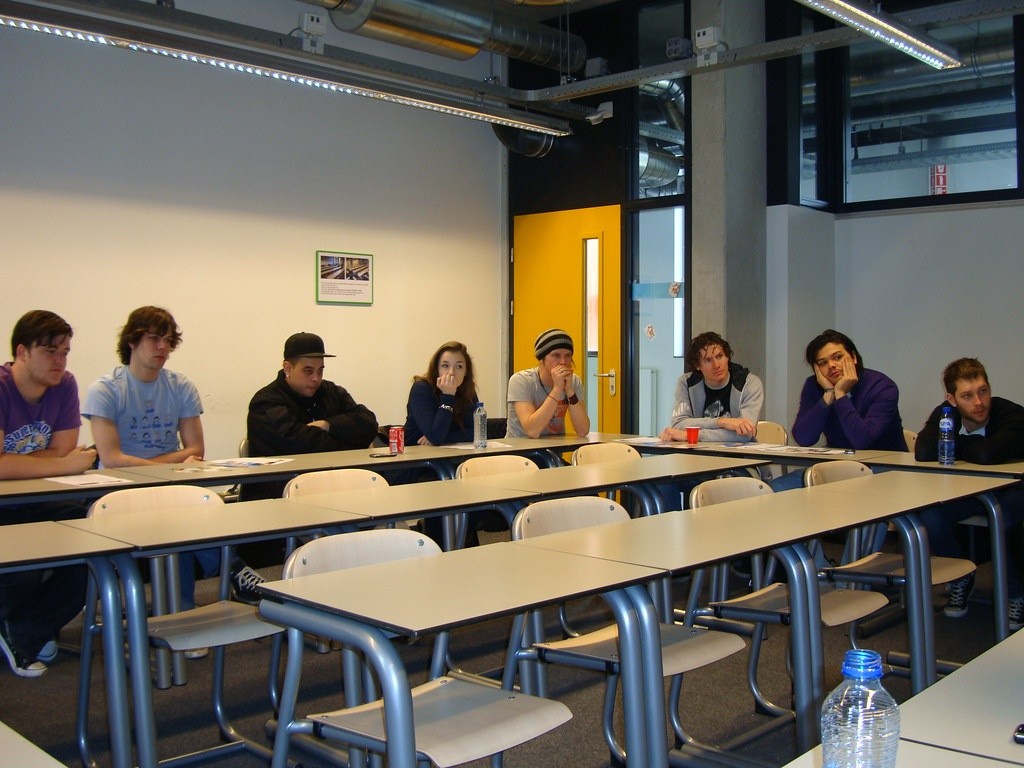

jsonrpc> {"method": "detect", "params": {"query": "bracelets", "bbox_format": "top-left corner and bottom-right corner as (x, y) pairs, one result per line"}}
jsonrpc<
(566, 393), (579, 405)
(548, 394), (560, 405)
(823, 387), (835, 394)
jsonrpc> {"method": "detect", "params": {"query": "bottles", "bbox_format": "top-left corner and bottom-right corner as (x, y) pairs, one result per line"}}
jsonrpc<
(819, 650), (900, 768)
(937, 407), (956, 464)
(472, 402), (487, 448)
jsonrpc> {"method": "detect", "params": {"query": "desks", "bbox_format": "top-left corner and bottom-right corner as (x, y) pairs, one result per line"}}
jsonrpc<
(696, 487), (928, 758)
(0, 467), (172, 690)
(601, 435), (900, 628)
(440, 430), (654, 540)
(858, 451), (1024, 640)
(54, 496), (373, 768)
(898, 628), (1024, 766)
(781, 736), (1024, 768)
(453, 451), (773, 698)
(254, 542), (669, 768)
(111, 444), (467, 687)
(509, 509), (817, 768)
(277, 477), (535, 768)
(0, 519), (138, 768)
(801, 469), (1022, 687)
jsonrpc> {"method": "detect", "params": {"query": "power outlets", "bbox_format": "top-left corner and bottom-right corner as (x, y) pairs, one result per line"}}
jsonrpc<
(297, 11), (329, 43)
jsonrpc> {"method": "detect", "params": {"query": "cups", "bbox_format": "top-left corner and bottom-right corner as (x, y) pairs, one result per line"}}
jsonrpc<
(685, 427), (700, 444)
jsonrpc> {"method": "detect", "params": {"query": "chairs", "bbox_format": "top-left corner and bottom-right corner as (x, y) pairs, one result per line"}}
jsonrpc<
(85, 440), (977, 768)
(886, 429), (918, 534)
(368, 424), (426, 534)
(754, 420), (789, 477)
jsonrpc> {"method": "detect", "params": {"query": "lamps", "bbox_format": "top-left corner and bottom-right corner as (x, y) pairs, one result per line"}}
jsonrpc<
(0, 0), (576, 137)
(795, 0), (968, 72)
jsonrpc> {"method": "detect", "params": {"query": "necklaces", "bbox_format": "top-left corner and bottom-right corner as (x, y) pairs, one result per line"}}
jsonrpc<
(537, 369), (549, 396)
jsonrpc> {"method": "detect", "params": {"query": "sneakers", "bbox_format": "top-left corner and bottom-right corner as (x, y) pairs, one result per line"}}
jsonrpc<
(229, 557), (276, 604)
(1008, 595), (1024, 630)
(0, 618), (59, 677)
(943, 572), (975, 618)
(183, 647), (209, 658)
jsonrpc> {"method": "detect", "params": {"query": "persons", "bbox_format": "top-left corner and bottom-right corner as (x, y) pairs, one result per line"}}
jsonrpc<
(504, 328), (590, 469)
(768, 329), (910, 492)
(401, 341), (480, 552)
(239, 332), (379, 501)
(914, 357), (1024, 630)
(658, 331), (764, 578)
(0, 309), (97, 676)
(80, 306), (276, 659)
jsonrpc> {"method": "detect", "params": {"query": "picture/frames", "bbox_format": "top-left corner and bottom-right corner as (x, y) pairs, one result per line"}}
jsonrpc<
(315, 250), (374, 305)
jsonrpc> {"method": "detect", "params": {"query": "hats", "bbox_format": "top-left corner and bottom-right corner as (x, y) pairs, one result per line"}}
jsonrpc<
(284, 332), (336, 358)
(534, 328), (574, 361)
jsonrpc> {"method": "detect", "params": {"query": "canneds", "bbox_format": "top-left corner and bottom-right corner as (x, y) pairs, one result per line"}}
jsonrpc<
(389, 426), (405, 454)
